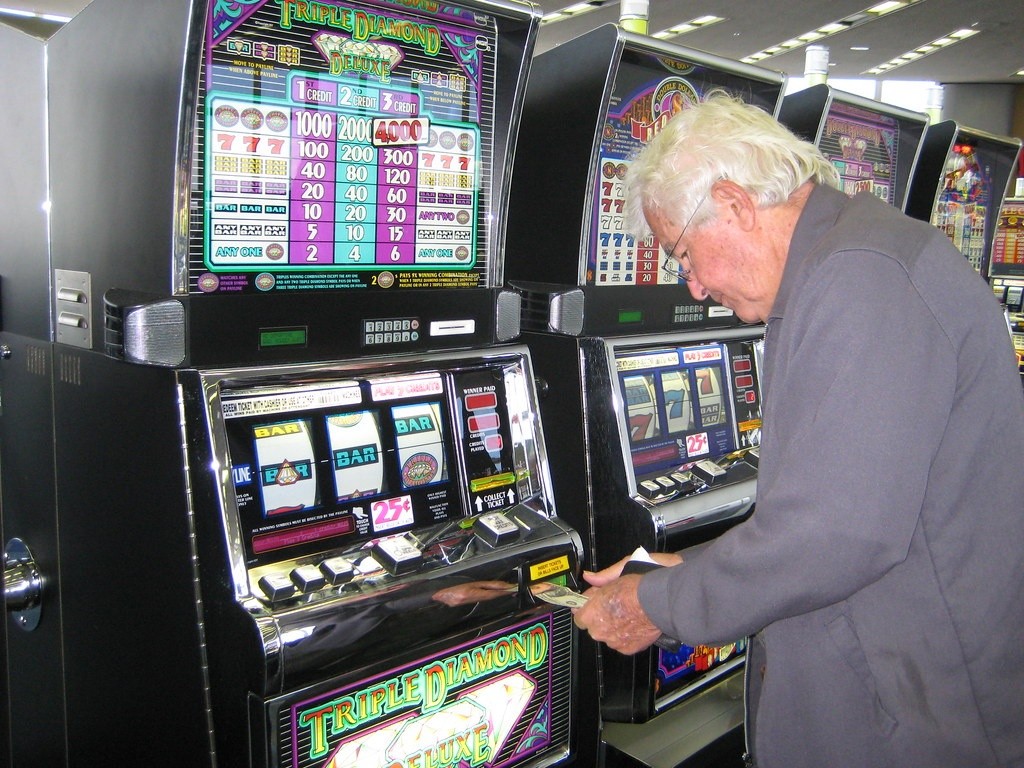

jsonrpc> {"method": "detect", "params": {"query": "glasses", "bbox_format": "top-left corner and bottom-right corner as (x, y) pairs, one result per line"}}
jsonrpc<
(661, 175), (728, 281)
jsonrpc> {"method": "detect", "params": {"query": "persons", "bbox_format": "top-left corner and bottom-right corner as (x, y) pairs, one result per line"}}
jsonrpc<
(285, 568), (518, 686)
(569, 89), (1021, 766)
